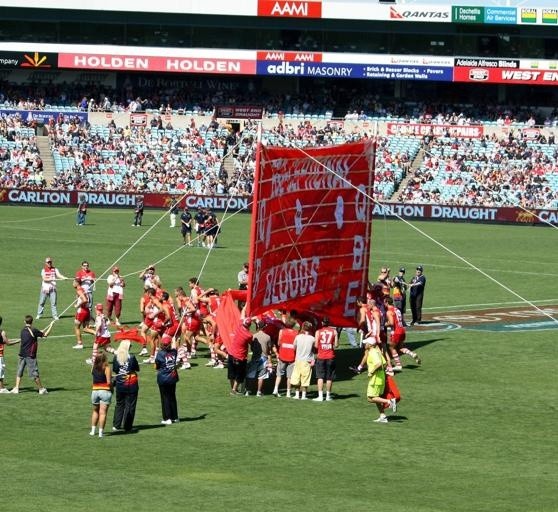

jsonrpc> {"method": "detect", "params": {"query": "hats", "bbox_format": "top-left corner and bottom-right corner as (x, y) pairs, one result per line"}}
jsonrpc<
(95, 303), (103, 310)
(112, 266), (119, 272)
(400, 267), (405, 272)
(362, 337), (376, 345)
(243, 317), (251, 325)
(45, 257), (52, 262)
(416, 266), (423, 271)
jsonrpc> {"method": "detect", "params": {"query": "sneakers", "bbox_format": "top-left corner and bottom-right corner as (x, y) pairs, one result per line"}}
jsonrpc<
(72, 345), (83, 349)
(230, 390), (333, 402)
(0, 387), (10, 394)
(161, 419), (172, 426)
(373, 416), (389, 424)
(348, 366), (361, 375)
(389, 398), (397, 413)
(39, 388), (48, 394)
(412, 353), (421, 364)
(10, 387), (19, 394)
(392, 366), (402, 371)
(138, 343), (225, 370)
(385, 369), (394, 376)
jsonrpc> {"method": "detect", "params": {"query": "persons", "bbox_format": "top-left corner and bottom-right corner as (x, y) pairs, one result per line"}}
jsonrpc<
(0, 318), (16, 393)
(0, 89), (557, 249)
(35, 257), (68, 320)
(73, 261), (340, 438)
(10, 315), (48, 395)
(344, 264), (424, 424)
